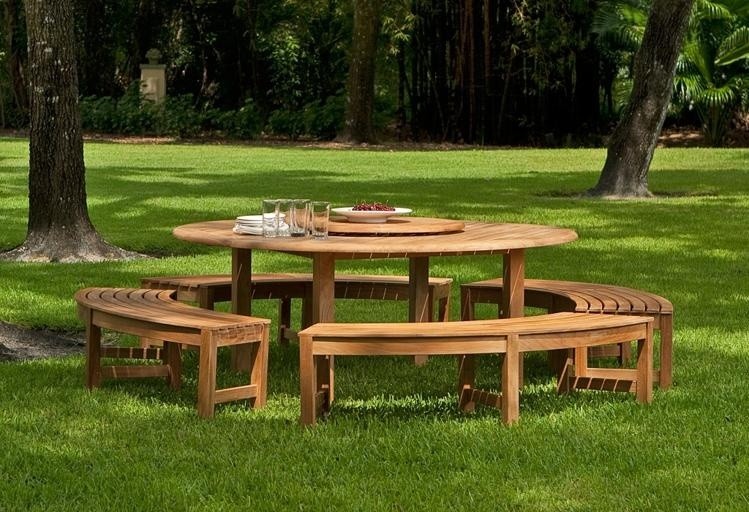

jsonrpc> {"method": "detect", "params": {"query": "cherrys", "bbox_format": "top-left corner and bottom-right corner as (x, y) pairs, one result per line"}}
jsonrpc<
(351, 202), (394, 211)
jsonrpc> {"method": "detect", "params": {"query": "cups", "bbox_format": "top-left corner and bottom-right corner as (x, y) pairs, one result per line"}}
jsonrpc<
(262, 199), (329, 241)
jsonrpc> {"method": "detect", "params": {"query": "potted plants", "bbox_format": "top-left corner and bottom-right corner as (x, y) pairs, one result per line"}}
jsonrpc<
(144, 48), (163, 65)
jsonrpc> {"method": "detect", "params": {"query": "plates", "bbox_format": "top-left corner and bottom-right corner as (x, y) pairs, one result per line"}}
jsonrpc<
(331, 207), (412, 223)
(233, 214), (289, 234)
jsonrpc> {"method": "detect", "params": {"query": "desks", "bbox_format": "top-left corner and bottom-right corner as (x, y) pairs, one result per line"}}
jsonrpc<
(173, 217), (580, 393)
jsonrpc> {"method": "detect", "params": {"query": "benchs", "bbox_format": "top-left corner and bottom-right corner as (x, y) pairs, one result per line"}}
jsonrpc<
(139, 274), (453, 346)
(73, 287), (271, 418)
(459, 278), (674, 391)
(297, 310), (653, 424)
